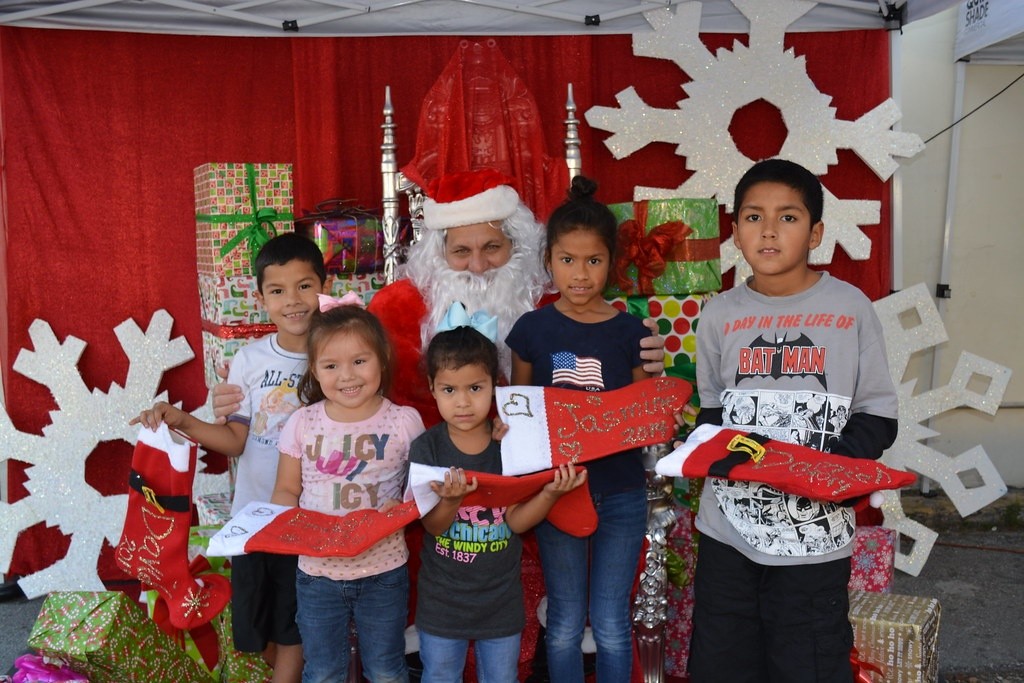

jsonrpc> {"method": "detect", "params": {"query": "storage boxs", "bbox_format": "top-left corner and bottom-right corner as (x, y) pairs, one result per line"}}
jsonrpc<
(191, 163), (724, 426)
(26, 521), (276, 683)
(848, 529), (941, 682)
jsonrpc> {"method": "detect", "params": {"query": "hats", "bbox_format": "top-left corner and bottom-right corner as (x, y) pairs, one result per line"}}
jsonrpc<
(400, 37), (571, 230)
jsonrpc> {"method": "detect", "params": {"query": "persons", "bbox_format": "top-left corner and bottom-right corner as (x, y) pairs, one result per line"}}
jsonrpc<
(129, 230), (326, 683)
(407, 325), (587, 683)
(269, 291), (425, 683)
(673, 160), (898, 683)
(491, 177), (695, 683)
(212, 162), (665, 683)
